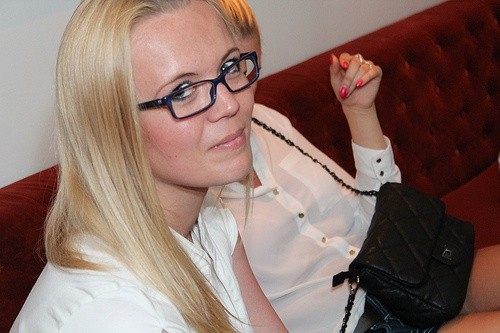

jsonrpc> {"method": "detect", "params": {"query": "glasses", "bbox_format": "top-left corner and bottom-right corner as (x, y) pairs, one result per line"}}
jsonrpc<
(137, 51), (260, 121)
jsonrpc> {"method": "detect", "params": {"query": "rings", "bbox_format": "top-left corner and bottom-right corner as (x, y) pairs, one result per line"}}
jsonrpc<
(365, 62), (372, 69)
(356, 54), (362, 62)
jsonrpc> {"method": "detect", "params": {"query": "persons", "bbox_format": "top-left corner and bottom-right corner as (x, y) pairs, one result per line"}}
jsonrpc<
(203, 0), (500, 333)
(0, 0), (287, 333)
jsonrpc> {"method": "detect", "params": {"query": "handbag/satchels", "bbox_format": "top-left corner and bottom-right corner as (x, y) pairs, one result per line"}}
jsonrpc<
(330, 181), (475, 328)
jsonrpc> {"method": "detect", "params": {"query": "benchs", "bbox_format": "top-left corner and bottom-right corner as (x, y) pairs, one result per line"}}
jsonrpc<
(0, 1), (500, 332)
